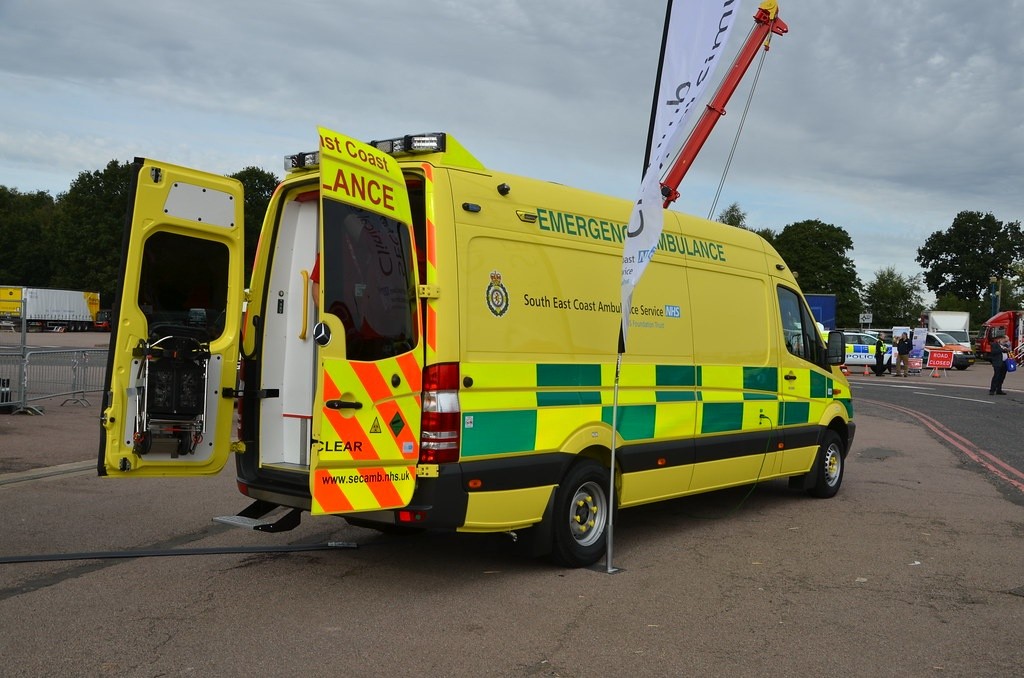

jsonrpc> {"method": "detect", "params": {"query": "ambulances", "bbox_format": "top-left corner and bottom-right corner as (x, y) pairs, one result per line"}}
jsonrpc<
(97, 131), (858, 567)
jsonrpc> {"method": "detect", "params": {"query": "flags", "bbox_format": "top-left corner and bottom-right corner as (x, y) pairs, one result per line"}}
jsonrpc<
(618, 0), (741, 353)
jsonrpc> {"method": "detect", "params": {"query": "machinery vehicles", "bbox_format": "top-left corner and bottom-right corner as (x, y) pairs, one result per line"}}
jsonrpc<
(659, 0), (788, 211)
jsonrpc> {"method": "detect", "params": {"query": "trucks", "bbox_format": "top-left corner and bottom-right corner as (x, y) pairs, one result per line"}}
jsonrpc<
(927, 311), (971, 349)
(974, 311), (1024, 363)
(96, 309), (112, 332)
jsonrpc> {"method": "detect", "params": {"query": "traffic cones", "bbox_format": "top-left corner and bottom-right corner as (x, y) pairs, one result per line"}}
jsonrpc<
(932, 367), (939, 377)
(863, 365), (869, 377)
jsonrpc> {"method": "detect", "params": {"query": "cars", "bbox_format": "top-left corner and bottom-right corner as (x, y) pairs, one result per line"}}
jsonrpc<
(822, 326), (975, 374)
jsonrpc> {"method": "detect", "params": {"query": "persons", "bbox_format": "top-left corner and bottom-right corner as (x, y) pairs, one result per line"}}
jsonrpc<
(874, 333), (886, 377)
(894, 332), (913, 377)
(988, 335), (1011, 395)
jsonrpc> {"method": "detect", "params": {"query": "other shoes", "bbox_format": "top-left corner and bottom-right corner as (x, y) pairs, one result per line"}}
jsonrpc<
(894, 373), (908, 377)
(876, 372), (885, 377)
(989, 390), (1006, 395)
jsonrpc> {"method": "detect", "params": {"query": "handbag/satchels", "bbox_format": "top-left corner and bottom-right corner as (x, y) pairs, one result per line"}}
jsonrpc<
(1005, 351), (1016, 372)
